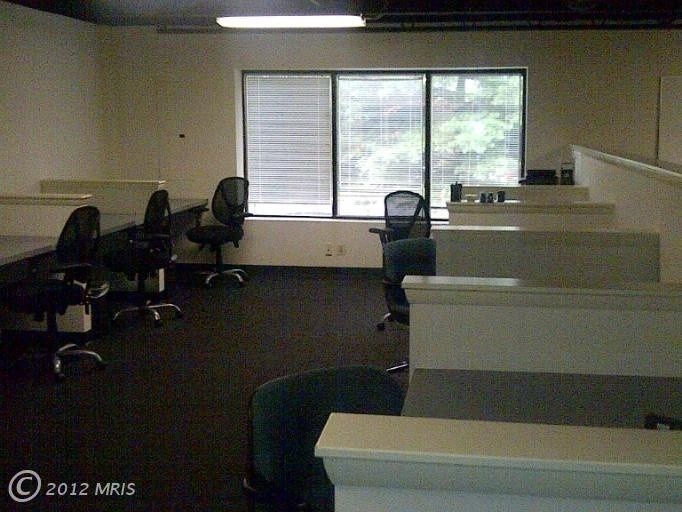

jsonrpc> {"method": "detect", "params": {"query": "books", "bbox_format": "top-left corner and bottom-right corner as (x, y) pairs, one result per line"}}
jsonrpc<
(515, 168), (559, 186)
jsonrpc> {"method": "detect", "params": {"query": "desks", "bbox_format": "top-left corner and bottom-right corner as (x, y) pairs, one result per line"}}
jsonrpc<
(244, 190), (435, 512)
(0, 177), (250, 379)
(399, 368), (682, 429)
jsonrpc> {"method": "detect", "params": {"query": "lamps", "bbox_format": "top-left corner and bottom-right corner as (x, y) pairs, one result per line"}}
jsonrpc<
(214, 9), (367, 29)
